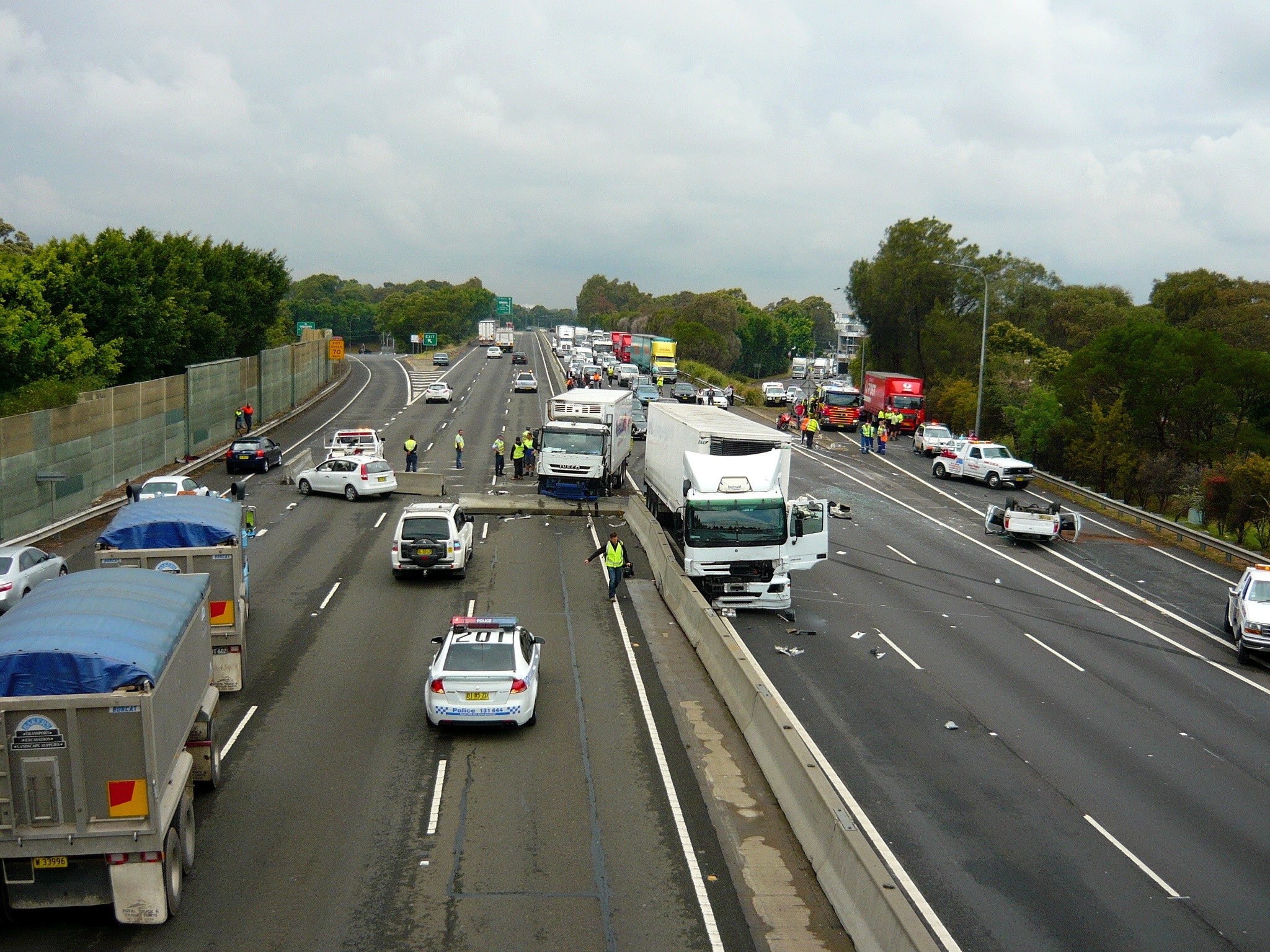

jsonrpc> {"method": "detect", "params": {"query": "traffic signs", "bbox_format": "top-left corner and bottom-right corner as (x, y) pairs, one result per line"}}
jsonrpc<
(423, 333), (438, 346)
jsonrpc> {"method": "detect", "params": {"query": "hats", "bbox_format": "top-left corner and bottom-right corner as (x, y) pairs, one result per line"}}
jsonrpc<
(526, 426), (531, 429)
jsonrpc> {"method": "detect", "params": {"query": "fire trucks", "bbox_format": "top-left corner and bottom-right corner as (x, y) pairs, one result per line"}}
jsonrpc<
(813, 380), (864, 433)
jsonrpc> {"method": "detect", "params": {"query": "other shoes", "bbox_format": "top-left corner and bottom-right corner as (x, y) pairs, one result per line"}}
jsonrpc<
(519, 476), (523, 479)
(867, 452), (870, 454)
(530, 472), (533, 476)
(456, 466), (464, 469)
(862, 452), (865, 454)
(876, 451), (880, 453)
(511, 477), (518, 480)
(523, 473), (529, 476)
(807, 445), (813, 449)
(889, 438), (892, 441)
(895, 438), (899, 440)
(610, 597), (615, 602)
(499, 472), (506, 475)
(496, 473), (502, 476)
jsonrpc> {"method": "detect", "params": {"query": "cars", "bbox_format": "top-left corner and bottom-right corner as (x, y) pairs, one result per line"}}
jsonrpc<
(423, 616), (546, 728)
(226, 436), (283, 474)
(425, 382), (454, 404)
(984, 496), (1081, 544)
(324, 428), (386, 467)
(0, 545), (69, 615)
(138, 475), (210, 501)
(432, 353), (450, 365)
(478, 319), (538, 393)
(295, 454), (398, 502)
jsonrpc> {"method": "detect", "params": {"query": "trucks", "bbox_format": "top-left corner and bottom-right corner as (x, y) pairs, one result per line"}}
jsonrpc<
(539, 325), (728, 440)
(761, 357), (837, 407)
(863, 370), (930, 432)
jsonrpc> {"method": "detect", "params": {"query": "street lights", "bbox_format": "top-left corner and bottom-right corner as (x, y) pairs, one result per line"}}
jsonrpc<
(349, 317), (360, 353)
(816, 307), (840, 380)
(931, 260), (989, 436)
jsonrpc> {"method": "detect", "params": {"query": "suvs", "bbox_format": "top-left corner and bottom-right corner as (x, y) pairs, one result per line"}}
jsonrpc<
(392, 502), (475, 580)
(1224, 563), (1270, 665)
(912, 422), (953, 456)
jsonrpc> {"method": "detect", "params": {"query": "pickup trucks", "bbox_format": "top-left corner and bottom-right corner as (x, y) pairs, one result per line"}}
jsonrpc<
(932, 436), (1035, 490)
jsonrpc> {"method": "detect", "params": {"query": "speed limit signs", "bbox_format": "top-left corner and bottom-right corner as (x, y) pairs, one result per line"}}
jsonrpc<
(329, 340), (344, 360)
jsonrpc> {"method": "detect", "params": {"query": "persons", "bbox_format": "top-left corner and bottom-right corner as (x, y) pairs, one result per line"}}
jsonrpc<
(878, 407), (903, 440)
(794, 401), (805, 429)
(362, 343), (365, 355)
(799, 397), (821, 448)
(724, 382), (735, 406)
(493, 426), (536, 480)
(608, 367), (614, 385)
(968, 430), (975, 437)
(403, 435), (417, 472)
(566, 369), (600, 390)
(808, 366), (810, 372)
(235, 403), (254, 437)
(707, 386), (715, 405)
(908, 409), (921, 438)
(455, 429), (465, 468)
(860, 421), (887, 455)
(697, 388), (703, 405)
(584, 533), (630, 602)
(657, 375), (664, 396)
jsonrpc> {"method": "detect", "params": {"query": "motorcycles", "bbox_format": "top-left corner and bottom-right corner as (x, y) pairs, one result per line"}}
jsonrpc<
(776, 412), (794, 431)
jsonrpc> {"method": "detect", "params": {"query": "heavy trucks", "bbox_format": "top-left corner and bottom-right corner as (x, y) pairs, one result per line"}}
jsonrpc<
(0, 569), (221, 924)
(93, 480), (256, 693)
(529, 388), (634, 501)
(642, 401), (829, 610)
